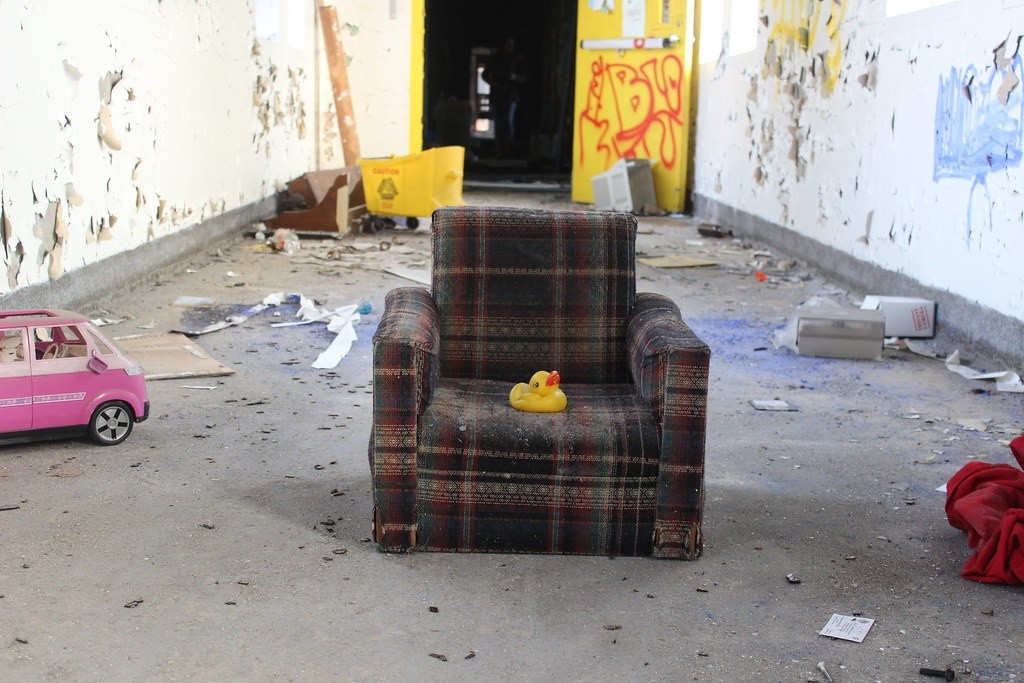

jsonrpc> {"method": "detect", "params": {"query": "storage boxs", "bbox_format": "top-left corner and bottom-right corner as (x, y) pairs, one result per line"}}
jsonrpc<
(796, 308), (887, 357)
(864, 295), (935, 338)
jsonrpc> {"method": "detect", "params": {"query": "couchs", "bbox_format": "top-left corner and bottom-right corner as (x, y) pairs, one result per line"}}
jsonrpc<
(369, 206), (710, 561)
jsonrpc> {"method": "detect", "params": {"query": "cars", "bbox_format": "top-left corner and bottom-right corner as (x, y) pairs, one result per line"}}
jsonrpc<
(0, 310), (151, 447)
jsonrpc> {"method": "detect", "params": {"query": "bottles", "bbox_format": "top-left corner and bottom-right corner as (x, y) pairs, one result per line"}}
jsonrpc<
(698, 223), (733, 239)
